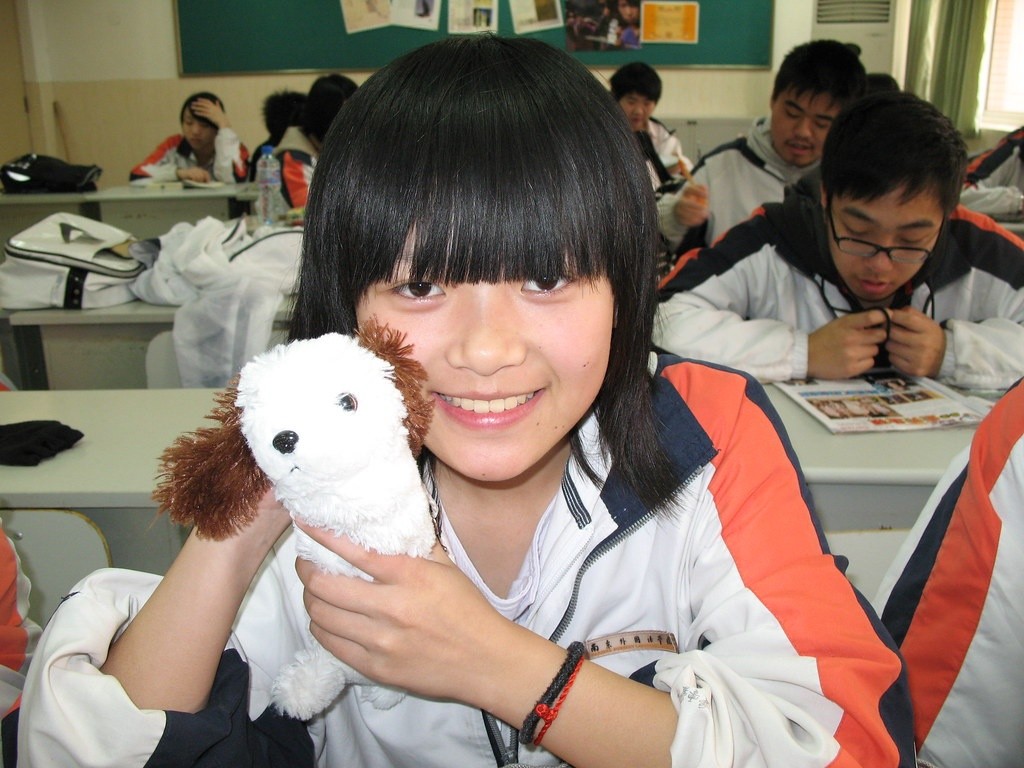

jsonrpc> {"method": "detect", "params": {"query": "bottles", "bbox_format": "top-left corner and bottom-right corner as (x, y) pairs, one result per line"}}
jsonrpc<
(256, 146), (281, 225)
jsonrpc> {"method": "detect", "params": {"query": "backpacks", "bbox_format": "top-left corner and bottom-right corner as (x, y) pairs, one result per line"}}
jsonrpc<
(0, 211), (157, 311)
(1, 155), (102, 197)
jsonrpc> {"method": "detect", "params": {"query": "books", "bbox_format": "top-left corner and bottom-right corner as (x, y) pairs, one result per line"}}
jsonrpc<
(145, 180), (225, 191)
(771, 364), (1006, 435)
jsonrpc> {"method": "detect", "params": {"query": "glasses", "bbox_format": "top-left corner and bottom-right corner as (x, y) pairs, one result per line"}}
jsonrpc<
(825, 191), (946, 264)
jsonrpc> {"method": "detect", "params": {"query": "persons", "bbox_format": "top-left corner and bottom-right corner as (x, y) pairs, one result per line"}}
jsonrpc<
(128, 92), (250, 185)
(651, 92), (1024, 389)
(653, 40), (1024, 257)
(16, 31), (919, 768)
(251, 73), (359, 211)
(608, 61), (694, 191)
(0, 523), (45, 768)
(869, 375), (1024, 768)
(566, 0), (641, 50)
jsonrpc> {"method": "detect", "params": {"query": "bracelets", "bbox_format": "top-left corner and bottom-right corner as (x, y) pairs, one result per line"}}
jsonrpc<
(519, 641), (586, 746)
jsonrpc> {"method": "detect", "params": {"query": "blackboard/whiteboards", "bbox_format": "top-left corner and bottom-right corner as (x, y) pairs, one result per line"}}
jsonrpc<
(170, 0), (774, 79)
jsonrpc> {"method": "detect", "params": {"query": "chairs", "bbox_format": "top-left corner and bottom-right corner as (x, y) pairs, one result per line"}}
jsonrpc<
(0, 510), (110, 629)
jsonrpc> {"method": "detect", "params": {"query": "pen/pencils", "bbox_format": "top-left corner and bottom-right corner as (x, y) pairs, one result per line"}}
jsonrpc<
(677, 156), (696, 188)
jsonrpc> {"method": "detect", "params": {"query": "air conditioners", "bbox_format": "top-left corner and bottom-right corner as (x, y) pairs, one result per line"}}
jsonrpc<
(810, 0), (900, 80)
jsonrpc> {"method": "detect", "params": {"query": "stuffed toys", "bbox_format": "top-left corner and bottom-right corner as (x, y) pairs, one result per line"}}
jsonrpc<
(147, 313), (435, 721)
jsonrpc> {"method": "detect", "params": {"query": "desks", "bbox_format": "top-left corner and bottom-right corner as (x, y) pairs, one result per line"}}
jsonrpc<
(0, 182), (1008, 603)
